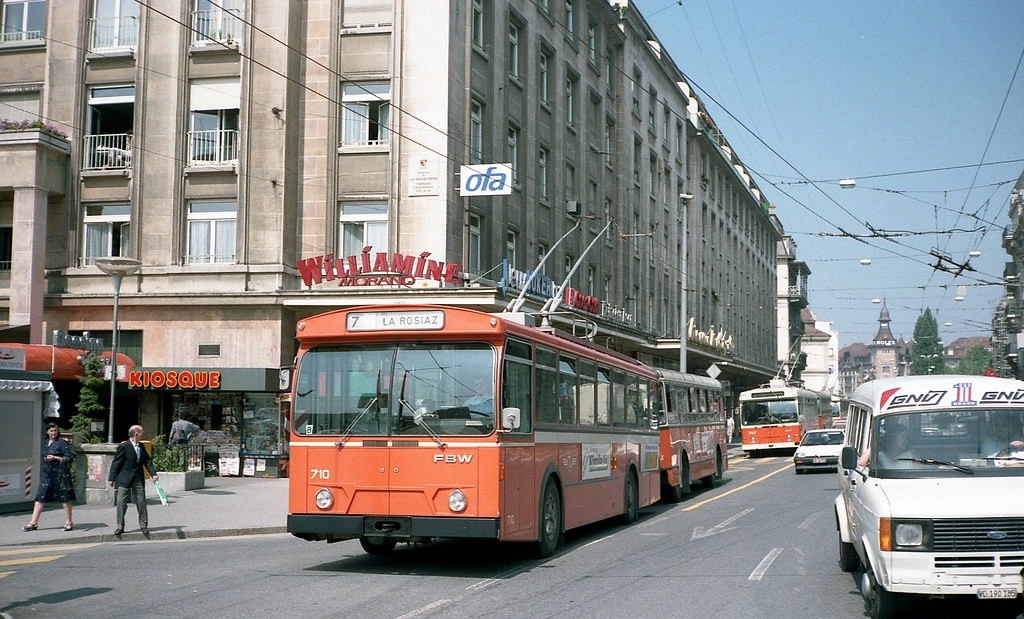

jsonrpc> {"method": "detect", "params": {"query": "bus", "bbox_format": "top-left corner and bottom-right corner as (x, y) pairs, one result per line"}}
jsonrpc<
(735, 387), (832, 459)
(278, 303), (729, 561)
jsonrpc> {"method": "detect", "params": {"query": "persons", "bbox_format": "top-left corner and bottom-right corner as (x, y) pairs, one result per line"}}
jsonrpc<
(167, 411), (199, 472)
(726, 415), (735, 444)
(857, 423), (921, 469)
(22, 423), (77, 531)
(108, 425), (158, 534)
(462, 373), (492, 420)
(981, 409), (1024, 457)
(106, 131), (133, 168)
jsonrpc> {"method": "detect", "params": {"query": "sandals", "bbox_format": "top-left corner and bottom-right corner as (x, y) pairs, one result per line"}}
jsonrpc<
(64, 522), (73, 531)
(21, 523), (38, 531)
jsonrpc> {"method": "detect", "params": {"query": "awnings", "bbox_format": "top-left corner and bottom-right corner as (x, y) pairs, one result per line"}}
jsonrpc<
(0, 343), (135, 382)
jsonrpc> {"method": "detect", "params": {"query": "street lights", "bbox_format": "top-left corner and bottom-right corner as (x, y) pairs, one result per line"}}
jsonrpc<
(93, 256), (143, 443)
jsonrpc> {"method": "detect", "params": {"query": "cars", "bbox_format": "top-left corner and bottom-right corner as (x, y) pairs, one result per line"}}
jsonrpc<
(831, 417), (847, 431)
(793, 430), (846, 477)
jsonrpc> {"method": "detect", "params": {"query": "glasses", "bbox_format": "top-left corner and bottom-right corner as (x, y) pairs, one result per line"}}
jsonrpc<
(474, 382), (487, 387)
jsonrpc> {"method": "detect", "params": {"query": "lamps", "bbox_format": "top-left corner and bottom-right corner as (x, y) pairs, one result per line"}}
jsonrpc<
(969, 251), (980, 257)
(839, 179), (856, 189)
(955, 297), (964, 301)
(860, 259), (871, 265)
(872, 298), (880, 304)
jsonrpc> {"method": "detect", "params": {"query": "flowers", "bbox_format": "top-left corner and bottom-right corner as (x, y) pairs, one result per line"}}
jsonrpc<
(0, 118), (69, 139)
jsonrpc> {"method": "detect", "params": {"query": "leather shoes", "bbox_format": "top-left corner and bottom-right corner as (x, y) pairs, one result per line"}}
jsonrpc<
(114, 528), (124, 535)
(140, 528), (149, 535)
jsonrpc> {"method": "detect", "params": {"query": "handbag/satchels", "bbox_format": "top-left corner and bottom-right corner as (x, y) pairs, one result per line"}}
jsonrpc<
(173, 421), (180, 442)
(59, 457), (72, 490)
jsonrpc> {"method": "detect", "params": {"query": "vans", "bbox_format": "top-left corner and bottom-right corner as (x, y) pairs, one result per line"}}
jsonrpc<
(834, 376), (1024, 619)
